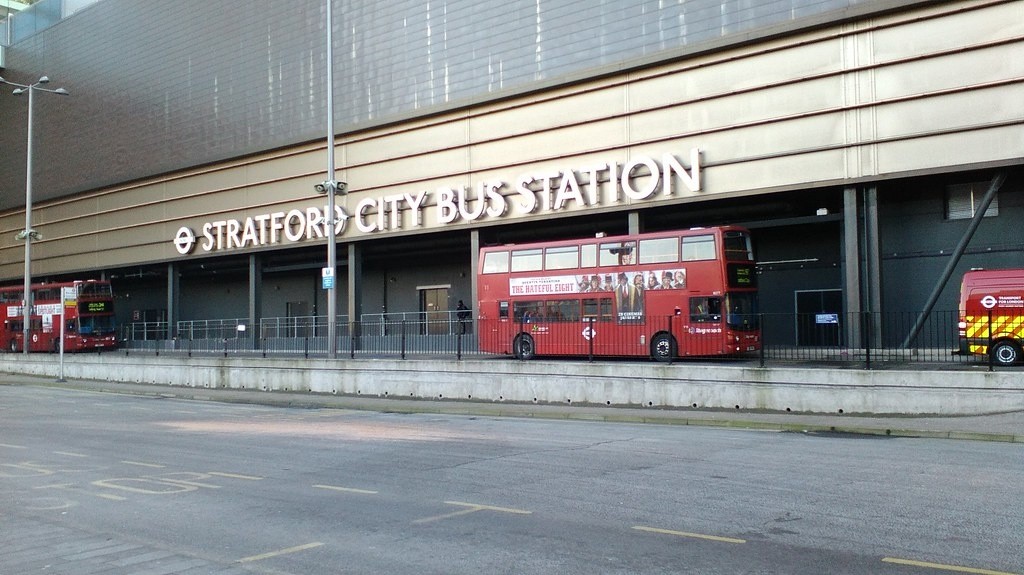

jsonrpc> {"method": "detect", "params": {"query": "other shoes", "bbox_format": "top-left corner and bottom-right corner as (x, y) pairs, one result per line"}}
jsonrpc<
(455, 333), (465, 335)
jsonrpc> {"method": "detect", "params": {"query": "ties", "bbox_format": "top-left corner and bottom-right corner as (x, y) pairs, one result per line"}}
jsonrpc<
(624, 287), (627, 298)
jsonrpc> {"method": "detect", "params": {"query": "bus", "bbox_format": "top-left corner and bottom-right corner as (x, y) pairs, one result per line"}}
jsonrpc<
(476, 225), (764, 362)
(951, 267), (1024, 365)
(0, 278), (118, 352)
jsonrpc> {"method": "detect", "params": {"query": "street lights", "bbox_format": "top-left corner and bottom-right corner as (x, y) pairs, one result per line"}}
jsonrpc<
(0, 74), (68, 354)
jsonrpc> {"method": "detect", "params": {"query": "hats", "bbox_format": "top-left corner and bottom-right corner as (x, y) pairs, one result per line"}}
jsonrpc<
(590, 276), (600, 282)
(662, 271), (674, 282)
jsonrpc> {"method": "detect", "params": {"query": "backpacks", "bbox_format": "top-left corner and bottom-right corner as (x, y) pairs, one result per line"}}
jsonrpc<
(466, 309), (469, 316)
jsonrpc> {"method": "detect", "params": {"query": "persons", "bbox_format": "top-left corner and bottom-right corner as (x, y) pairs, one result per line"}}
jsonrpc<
(585, 273), (674, 313)
(527, 312), (538, 323)
(455, 299), (467, 335)
